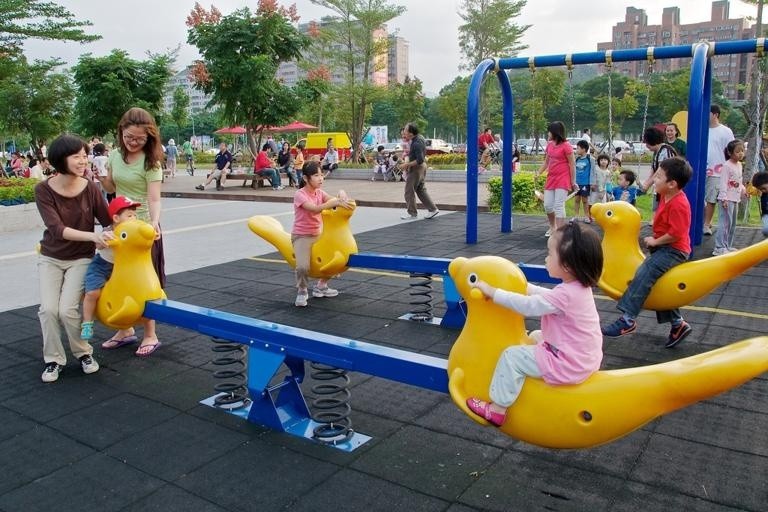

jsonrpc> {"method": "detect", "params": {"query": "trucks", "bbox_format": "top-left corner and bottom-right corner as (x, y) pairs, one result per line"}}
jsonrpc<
(291, 130), (352, 161)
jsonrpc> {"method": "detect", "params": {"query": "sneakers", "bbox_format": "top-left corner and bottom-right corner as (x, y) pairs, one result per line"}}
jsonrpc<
(728, 248), (738, 252)
(290, 183), (300, 187)
(196, 185), (204, 190)
(312, 287), (338, 297)
(400, 214), (418, 220)
(466, 397), (506, 427)
(372, 176), (376, 182)
(273, 186), (283, 190)
(80, 354), (99, 375)
(712, 248), (730, 256)
(569, 216), (579, 222)
(217, 186), (224, 190)
(545, 228), (556, 237)
(80, 322), (94, 340)
(702, 224), (712, 236)
(384, 177), (389, 182)
(424, 209), (439, 219)
(665, 321), (691, 348)
(295, 291), (309, 307)
(280, 185), (286, 189)
(41, 362), (62, 382)
(584, 218), (591, 224)
(601, 317), (637, 339)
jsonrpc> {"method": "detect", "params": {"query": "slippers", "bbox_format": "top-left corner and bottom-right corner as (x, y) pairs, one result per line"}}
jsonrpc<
(135, 342), (161, 357)
(102, 336), (137, 349)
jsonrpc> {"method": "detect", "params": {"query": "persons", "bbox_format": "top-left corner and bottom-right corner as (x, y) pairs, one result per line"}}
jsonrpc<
(707, 140), (751, 257)
(459, 222), (609, 427)
(31, 132), (114, 383)
(290, 160), (355, 307)
(396, 121), (439, 220)
(165, 134), (305, 191)
(598, 155), (693, 349)
(534, 121), (580, 238)
(320, 138), (338, 178)
(93, 108), (165, 360)
(372, 141), (409, 182)
(79, 196), (140, 342)
(6, 136), (115, 184)
(701, 101), (735, 237)
(494, 134), (521, 163)
(575, 129), (646, 222)
(751, 171), (768, 241)
(477, 127), (495, 166)
(638, 127), (678, 222)
(662, 122), (686, 157)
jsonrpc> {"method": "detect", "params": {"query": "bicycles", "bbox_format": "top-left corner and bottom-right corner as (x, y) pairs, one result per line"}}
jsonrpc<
(181, 154), (196, 177)
(473, 139), (505, 176)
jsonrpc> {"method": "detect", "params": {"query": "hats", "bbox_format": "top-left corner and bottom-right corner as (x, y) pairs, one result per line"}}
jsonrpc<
(108, 196), (142, 220)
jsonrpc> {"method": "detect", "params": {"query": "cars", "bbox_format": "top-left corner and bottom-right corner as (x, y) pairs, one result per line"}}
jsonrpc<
(509, 134), (649, 156)
(365, 140), (403, 157)
(452, 142), (466, 153)
(397, 137), (453, 157)
(741, 141), (748, 151)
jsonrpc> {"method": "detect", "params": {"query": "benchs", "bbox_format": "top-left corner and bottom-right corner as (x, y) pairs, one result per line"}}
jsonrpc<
(207, 172), (287, 189)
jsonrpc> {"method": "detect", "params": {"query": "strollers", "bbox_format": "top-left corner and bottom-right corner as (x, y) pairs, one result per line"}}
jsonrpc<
(385, 157), (407, 183)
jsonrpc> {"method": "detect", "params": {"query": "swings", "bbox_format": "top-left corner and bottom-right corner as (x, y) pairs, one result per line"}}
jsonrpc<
(603, 61), (655, 213)
(529, 65), (580, 202)
